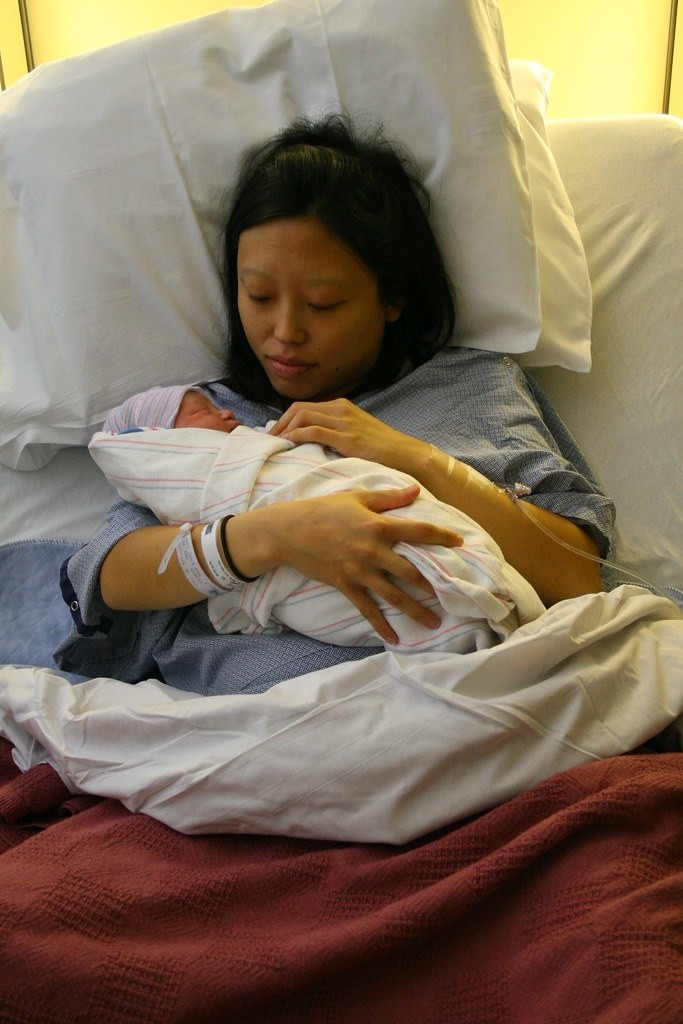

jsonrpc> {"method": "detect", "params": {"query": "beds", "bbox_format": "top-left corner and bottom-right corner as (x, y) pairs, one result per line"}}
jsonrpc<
(0, 106), (683, 614)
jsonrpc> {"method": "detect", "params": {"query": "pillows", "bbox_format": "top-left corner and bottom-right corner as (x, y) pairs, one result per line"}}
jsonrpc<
(0, 1), (591, 473)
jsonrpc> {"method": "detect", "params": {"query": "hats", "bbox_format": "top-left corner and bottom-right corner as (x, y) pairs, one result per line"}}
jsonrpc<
(102, 386), (215, 436)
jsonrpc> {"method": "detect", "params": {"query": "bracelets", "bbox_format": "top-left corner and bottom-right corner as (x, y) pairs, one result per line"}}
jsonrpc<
(156, 513), (262, 594)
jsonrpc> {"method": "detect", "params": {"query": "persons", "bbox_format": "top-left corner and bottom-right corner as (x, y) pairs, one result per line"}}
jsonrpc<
(89, 385), (547, 655)
(50, 120), (616, 695)
